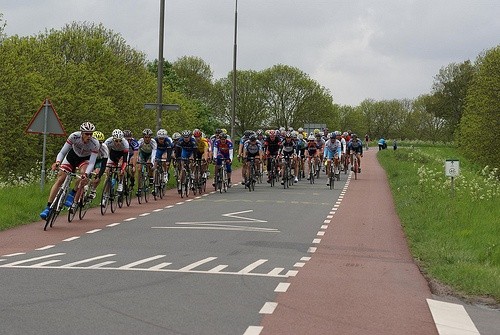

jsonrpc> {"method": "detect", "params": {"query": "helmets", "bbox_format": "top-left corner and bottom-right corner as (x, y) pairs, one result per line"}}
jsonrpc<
(123, 130), (133, 139)
(93, 131), (104, 141)
(243, 127), (357, 141)
(112, 129), (124, 139)
(142, 129), (228, 140)
(80, 122), (97, 132)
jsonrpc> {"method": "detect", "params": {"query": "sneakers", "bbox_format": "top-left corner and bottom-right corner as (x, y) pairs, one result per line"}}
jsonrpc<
(40, 208), (49, 218)
(64, 192), (74, 206)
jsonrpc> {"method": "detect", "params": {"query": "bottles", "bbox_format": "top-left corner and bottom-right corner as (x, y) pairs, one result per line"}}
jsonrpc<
(112, 178), (116, 185)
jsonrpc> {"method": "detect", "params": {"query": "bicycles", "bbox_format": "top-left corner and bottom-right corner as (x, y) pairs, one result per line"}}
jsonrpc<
(100, 165), (123, 216)
(243, 157), (260, 192)
(215, 158), (231, 193)
(44, 163), (85, 231)
(175, 157), (195, 198)
(135, 161), (153, 204)
(67, 172), (97, 223)
(207, 159), (220, 191)
(118, 163), (135, 208)
(172, 158), (209, 196)
(324, 156), (339, 190)
(236, 153), (323, 189)
(152, 158), (169, 201)
(341, 150), (361, 180)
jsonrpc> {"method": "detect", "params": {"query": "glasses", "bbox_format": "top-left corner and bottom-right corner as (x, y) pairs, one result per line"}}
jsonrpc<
(145, 136), (150, 138)
(83, 133), (93, 136)
(115, 140), (122, 142)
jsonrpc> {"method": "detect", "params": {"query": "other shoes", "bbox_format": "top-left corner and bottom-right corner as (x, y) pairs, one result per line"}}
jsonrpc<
(99, 196), (107, 207)
(117, 184), (123, 192)
(89, 190), (97, 199)
(294, 176), (298, 183)
(68, 202), (77, 214)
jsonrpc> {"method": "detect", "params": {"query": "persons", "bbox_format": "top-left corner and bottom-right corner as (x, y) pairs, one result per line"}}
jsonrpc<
(379, 138), (397, 151)
(39, 122), (369, 219)
